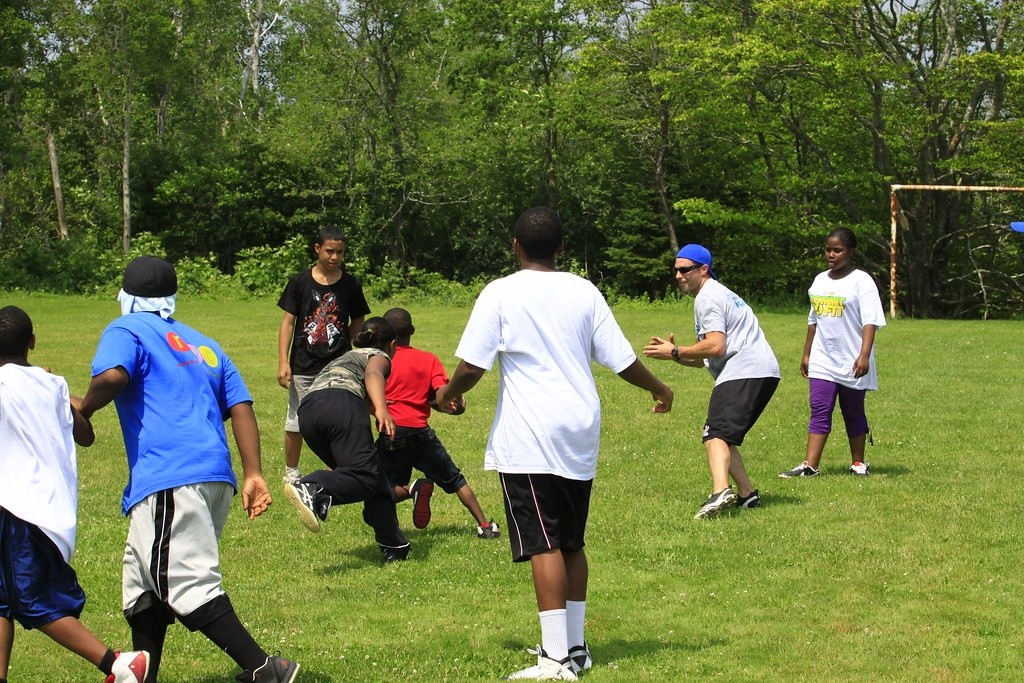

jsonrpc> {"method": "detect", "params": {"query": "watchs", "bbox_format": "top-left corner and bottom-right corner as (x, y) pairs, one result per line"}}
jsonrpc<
(671, 346), (678, 357)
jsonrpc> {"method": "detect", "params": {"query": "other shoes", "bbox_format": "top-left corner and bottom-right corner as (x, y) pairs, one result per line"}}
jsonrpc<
(283, 469), (301, 485)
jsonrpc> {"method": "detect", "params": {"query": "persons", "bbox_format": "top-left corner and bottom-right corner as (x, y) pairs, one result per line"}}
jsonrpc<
(644, 244), (781, 519)
(437, 206), (673, 679)
(283, 317), (411, 563)
(68, 255), (301, 683)
(0, 306), (150, 683)
(276, 224), (371, 481)
(373, 308), (501, 539)
(778, 227), (886, 477)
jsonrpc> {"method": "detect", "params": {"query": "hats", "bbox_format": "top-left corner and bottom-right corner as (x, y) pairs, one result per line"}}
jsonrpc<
(122, 256), (178, 298)
(1011, 222), (1024, 233)
(676, 244), (718, 279)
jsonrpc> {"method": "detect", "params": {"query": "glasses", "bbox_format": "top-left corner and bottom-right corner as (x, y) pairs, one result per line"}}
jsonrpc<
(673, 264), (700, 274)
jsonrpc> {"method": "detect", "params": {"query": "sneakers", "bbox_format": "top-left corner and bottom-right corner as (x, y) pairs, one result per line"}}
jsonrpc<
(283, 482), (332, 533)
(478, 518), (501, 540)
(104, 650), (150, 683)
(508, 644), (579, 682)
(849, 461), (870, 477)
(735, 489), (761, 507)
(410, 479), (434, 528)
(779, 461), (820, 480)
(568, 640), (593, 675)
(235, 650), (300, 683)
(377, 544), (412, 564)
(694, 485), (738, 520)
